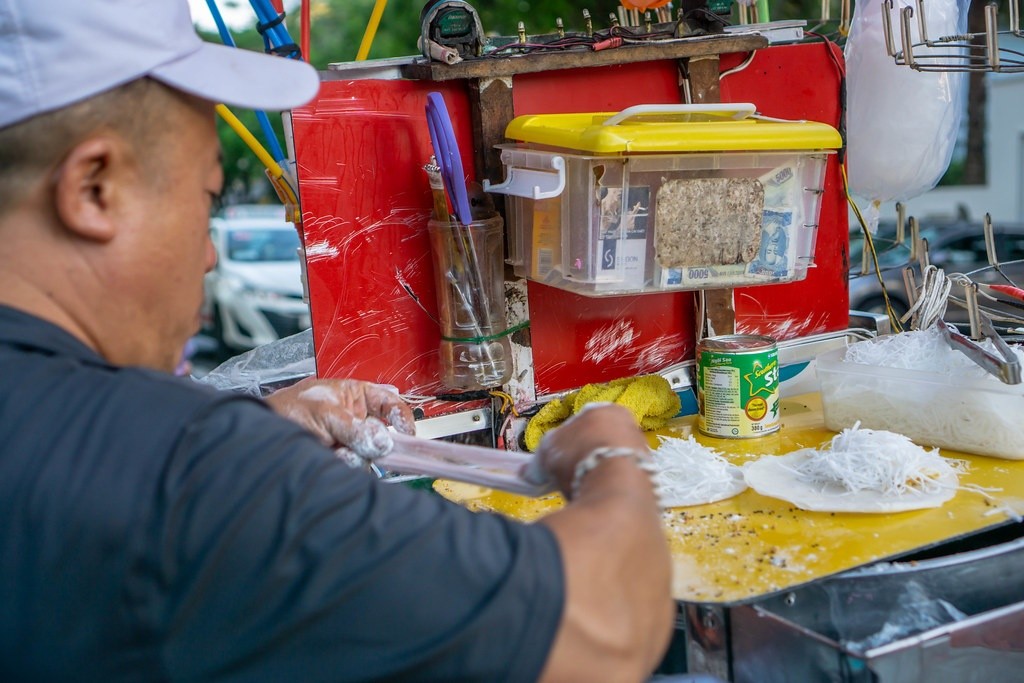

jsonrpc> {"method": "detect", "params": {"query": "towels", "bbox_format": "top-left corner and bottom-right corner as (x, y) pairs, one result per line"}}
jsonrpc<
(523, 373), (682, 454)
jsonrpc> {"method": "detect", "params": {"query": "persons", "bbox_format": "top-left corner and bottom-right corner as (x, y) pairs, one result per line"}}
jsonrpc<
(0, 0), (677, 683)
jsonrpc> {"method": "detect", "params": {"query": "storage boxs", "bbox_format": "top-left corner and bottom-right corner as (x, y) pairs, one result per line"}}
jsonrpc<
(478, 102), (844, 299)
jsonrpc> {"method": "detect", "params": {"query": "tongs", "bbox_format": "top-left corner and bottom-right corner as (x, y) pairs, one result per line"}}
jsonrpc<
(936, 309), (1023, 385)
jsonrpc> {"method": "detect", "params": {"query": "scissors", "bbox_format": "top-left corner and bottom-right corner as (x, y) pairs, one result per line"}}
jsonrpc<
(424, 91), (494, 340)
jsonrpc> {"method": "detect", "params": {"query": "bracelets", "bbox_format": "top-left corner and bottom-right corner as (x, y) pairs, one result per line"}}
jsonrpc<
(567, 444), (664, 507)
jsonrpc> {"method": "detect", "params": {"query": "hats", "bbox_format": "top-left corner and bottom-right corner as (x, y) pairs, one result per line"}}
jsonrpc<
(0, 0), (320, 130)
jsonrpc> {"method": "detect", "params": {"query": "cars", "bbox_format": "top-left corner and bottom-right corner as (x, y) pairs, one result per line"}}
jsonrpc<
(847, 218), (1023, 346)
(192, 217), (313, 367)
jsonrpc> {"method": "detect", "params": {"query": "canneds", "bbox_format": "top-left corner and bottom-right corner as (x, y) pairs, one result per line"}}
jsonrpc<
(697, 336), (781, 440)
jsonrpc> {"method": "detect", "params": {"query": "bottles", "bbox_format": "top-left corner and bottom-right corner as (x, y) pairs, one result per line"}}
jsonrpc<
(430, 210), (513, 392)
